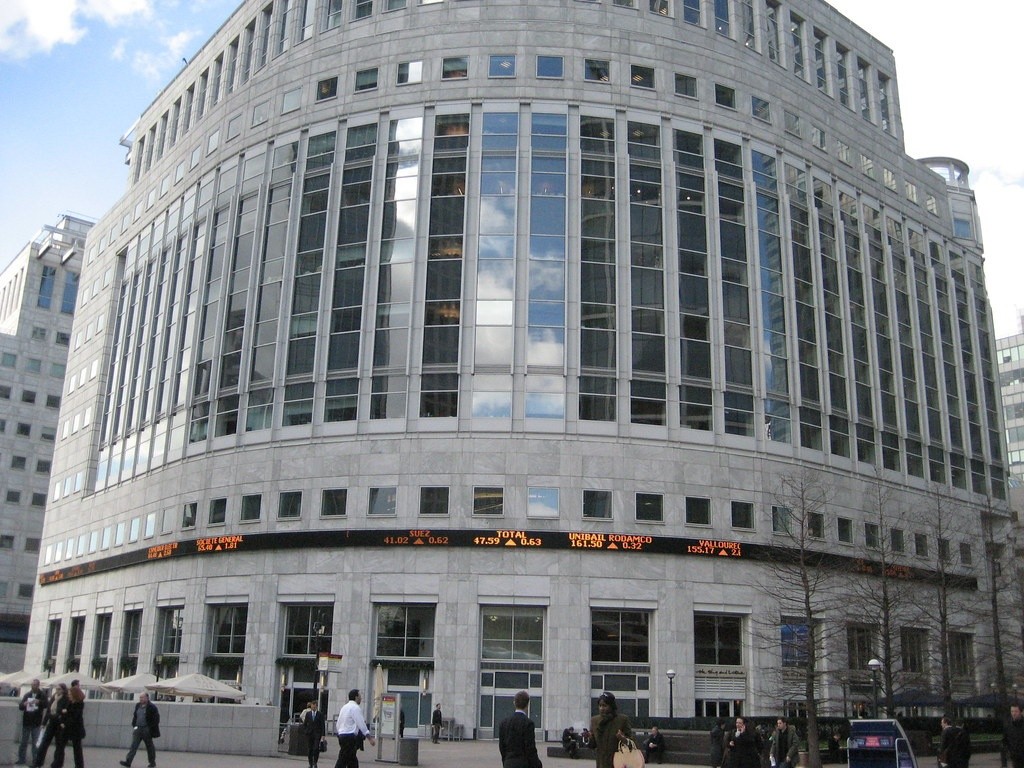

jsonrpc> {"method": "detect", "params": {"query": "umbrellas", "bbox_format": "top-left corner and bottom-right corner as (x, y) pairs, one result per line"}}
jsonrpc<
(876, 689), (953, 716)
(373, 663), (387, 738)
(955, 693), (1024, 718)
(0, 669), (245, 702)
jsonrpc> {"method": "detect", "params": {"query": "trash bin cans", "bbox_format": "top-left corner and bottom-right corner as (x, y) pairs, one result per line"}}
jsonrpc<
(399, 737), (419, 765)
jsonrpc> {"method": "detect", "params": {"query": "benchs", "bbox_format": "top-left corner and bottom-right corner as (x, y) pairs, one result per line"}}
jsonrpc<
(547, 729), (730, 766)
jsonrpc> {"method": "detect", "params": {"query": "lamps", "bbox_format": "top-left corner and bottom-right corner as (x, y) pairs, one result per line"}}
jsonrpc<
(490, 616), (498, 622)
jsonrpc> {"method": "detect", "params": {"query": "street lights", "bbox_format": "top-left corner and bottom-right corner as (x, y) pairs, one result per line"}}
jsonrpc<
(666, 669), (676, 718)
(1012, 684), (1018, 702)
(990, 683), (998, 718)
(842, 677), (849, 720)
(45, 658), (54, 695)
(869, 658), (880, 716)
(153, 652), (164, 703)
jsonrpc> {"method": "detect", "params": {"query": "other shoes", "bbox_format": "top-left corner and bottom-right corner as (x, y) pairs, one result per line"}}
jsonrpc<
(148, 763), (156, 768)
(120, 761), (131, 768)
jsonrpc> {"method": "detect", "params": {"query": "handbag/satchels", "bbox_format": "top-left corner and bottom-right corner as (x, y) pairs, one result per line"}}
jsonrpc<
(319, 739), (327, 752)
(613, 738), (645, 768)
(937, 750), (949, 768)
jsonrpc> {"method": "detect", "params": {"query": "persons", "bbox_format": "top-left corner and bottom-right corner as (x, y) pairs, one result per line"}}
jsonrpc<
(28, 679), (86, 768)
(562, 727), (593, 759)
(266, 701), (273, 707)
(119, 692), (160, 768)
(334, 688), (375, 768)
(432, 703), (442, 744)
(644, 727), (664, 764)
(829, 733), (848, 763)
(937, 703), (972, 768)
(300, 702), (325, 768)
(710, 716), (800, 768)
(499, 691), (543, 768)
(178, 697), (205, 703)
(582, 692), (638, 768)
(15, 680), (48, 765)
(1001, 703), (1024, 768)
(399, 703), (404, 738)
